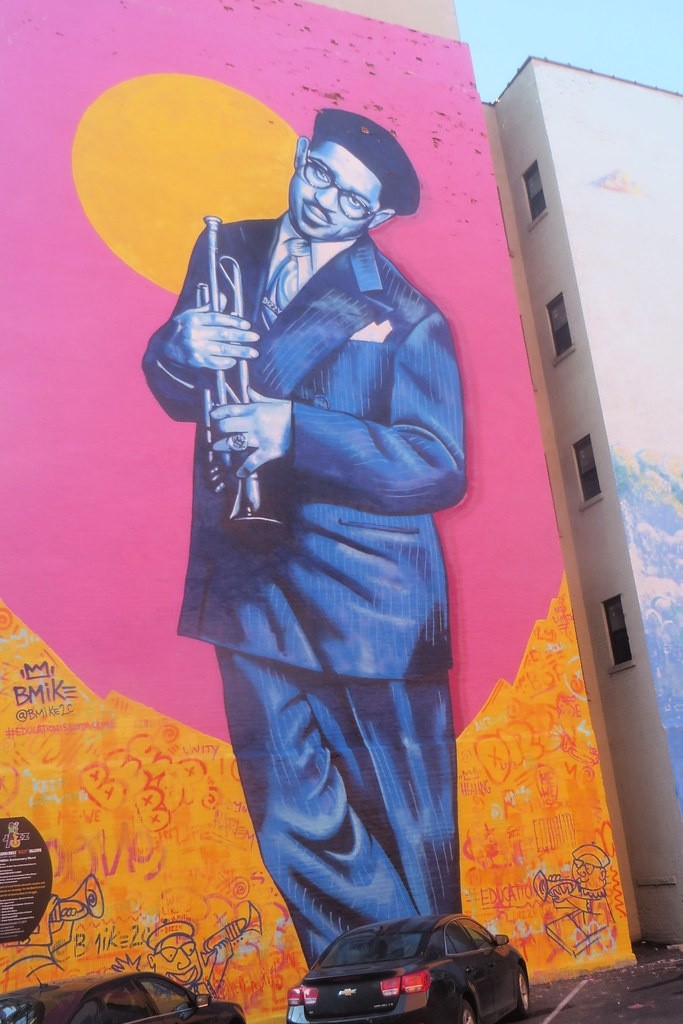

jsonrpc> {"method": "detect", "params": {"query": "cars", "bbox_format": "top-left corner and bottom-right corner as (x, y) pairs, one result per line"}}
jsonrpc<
(0, 970), (246, 1024)
(286, 914), (531, 1024)
